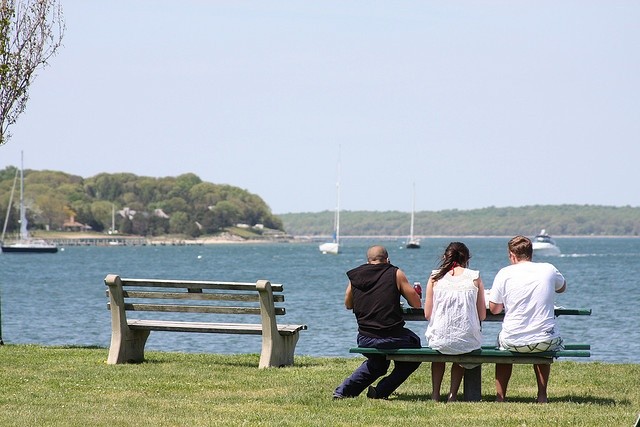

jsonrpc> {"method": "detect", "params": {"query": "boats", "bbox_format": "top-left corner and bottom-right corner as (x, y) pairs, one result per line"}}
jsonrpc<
(532, 229), (560, 254)
(406, 240), (420, 248)
(319, 242), (341, 254)
(1, 239), (57, 253)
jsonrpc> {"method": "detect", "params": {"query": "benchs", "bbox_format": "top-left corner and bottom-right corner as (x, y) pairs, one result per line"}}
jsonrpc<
(105, 274), (307, 370)
(350, 343), (592, 398)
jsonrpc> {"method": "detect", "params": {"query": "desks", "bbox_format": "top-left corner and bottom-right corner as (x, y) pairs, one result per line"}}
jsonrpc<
(401, 307), (593, 398)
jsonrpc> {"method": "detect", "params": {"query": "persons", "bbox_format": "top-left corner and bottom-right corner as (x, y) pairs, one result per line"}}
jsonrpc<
(332, 245), (421, 399)
(489, 235), (566, 403)
(425, 241), (487, 400)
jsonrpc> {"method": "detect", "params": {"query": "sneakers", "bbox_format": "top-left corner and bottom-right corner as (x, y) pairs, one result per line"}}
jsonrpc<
(367, 385), (388, 400)
(332, 390), (354, 400)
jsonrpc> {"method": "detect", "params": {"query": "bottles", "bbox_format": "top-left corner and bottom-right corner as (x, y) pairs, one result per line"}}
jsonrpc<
(413, 281), (423, 306)
